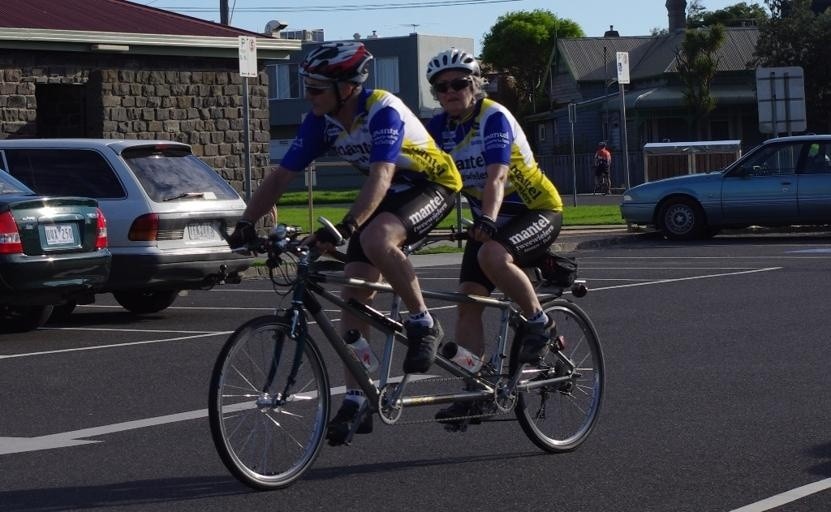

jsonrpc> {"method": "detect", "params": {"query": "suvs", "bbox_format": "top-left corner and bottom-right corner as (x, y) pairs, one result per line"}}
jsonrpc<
(0, 135), (254, 319)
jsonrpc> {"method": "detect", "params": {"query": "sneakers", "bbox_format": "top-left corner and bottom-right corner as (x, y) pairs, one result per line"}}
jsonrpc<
(435, 397), (483, 425)
(518, 314), (557, 364)
(402, 313), (444, 372)
(326, 400), (372, 439)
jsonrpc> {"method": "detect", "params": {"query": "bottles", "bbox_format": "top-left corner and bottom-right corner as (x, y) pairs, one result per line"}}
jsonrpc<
(342, 328), (381, 374)
(441, 341), (482, 376)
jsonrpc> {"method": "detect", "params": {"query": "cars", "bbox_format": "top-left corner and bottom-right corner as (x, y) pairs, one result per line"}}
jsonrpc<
(618, 132), (829, 244)
(0, 167), (117, 340)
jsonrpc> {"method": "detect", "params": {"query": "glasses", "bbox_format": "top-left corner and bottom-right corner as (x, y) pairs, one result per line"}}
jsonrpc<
(434, 77), (472, 94)
(304, 81), (337, 94)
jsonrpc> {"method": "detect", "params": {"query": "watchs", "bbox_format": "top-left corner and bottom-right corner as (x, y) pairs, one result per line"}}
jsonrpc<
(342, 211), (362, 234)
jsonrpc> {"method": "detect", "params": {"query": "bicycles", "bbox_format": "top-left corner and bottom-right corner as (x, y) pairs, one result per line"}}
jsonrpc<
(592, 157), (614, 197)
(206, 219), (608, 493)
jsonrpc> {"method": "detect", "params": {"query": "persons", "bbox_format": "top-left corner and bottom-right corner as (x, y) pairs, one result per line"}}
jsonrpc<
(592, 141), (612, 195)
(417, 47), (567, 427)
(231, 38), (465, 448)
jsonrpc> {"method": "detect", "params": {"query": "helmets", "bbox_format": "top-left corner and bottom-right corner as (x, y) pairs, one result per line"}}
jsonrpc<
(426, 47), (481, 84)
(301, 42), (375, 86)
